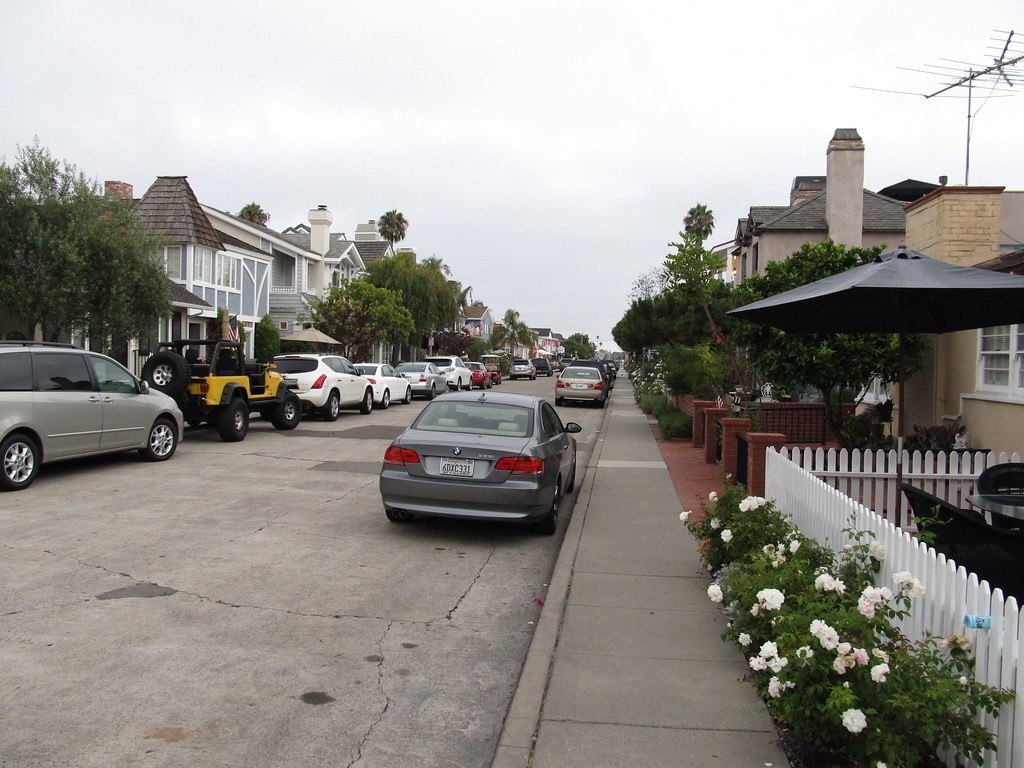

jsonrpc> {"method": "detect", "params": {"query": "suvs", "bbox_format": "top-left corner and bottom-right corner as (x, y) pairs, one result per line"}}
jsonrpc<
(423, 354), (474, 391)
(141, 339), (303, 442)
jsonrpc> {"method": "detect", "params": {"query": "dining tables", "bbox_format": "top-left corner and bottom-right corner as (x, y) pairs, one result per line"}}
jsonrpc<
(964, 493), (1024, 525)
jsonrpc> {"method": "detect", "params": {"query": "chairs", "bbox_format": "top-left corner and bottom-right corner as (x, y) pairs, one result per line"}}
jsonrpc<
(896, 480), (1024, 611)
(975, 463), (1024, 506)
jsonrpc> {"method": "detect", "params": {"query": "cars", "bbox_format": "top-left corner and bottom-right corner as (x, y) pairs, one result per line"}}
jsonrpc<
(570, 360), (621, 398)
(463, 361), (492, 389)
(559, 358), (573, 372)
(509, 359), (537, 381)
(0, 339), (184, 493)
(394, 362), (449, 401)
(275, 353), (373, 421)
(352, 362), (412, 408)
(531, 358), (553, 377)
(379, 392), (582, 535)
(482, 355), (502, 385)
(554, 367), (607, 408)
